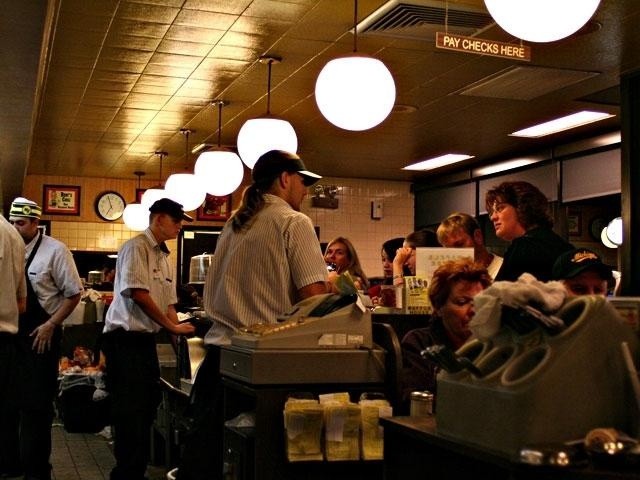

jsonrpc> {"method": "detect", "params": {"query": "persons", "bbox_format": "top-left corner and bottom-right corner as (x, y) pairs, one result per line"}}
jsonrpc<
(177, 147), (332, 480)
(322, 230), (442, 309)
(10, 195), (87, 479)
(97, 197), (198, 479)
(556, 247), (612, 296)
(437, 212), (505, 281)
(401, 257), (495, 410)
(485, 180), (575, 282)
(0, 211), (29, 479)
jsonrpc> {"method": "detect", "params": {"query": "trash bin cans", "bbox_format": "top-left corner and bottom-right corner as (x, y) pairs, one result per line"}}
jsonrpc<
(59, 372), (108, 434)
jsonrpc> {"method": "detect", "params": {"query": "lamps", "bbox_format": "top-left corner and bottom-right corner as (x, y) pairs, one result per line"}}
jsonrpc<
(314, 0), (396, 131)
(122, 56), (299, 232)
(312, 185), (339, 209)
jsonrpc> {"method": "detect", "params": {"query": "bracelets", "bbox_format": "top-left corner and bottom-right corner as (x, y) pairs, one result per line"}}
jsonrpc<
(48, 318), (61, 327)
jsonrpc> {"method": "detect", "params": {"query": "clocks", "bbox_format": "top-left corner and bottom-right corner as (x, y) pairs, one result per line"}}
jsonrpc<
(588, 216), (609, 241)
(96, 190), (126, 222)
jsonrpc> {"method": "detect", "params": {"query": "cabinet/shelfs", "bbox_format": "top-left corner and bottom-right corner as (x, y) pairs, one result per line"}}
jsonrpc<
(220, 378), (383, 480)
(379, 413), (640, 480)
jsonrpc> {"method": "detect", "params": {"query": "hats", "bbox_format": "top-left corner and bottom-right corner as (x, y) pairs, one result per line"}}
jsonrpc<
(551, 248), (613, 280)
(9, 197), (43, 220)
(252, 150), (323, 185)
(150, 198), (195, 223)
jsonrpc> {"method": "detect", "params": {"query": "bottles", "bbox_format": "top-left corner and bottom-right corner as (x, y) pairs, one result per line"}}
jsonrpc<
(409, 388), (436, 430)
(83, 294), (114, 324)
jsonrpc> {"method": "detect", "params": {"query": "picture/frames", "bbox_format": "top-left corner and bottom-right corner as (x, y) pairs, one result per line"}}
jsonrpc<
(568, 211), (583, 236)
(42, 183), (82, 216)
